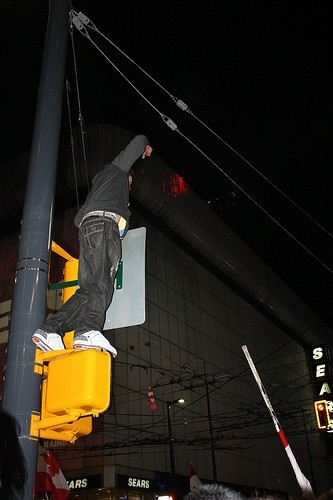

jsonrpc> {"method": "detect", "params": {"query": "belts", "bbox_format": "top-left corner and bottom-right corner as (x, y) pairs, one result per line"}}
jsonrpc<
(78, 211), (119, 226)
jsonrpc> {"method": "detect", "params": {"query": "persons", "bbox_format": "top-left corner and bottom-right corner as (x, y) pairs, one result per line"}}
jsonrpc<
(31, 134), (155, 358)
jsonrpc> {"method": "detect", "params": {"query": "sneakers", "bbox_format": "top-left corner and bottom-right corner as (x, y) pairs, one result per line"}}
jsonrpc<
(72, 330), (117, 358)
(31, 329), (65, 351)
